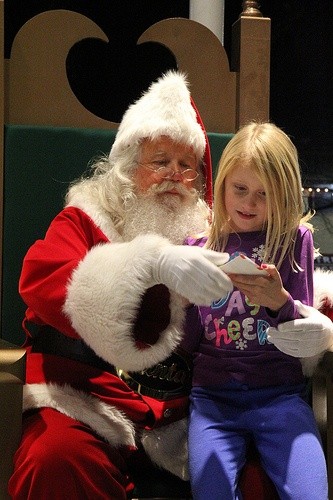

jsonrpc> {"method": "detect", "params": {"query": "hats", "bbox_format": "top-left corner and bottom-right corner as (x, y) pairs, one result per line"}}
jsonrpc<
(109, 71), (207, 173)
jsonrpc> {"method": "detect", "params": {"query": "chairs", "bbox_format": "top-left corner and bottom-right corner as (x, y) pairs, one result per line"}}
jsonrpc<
(0, 125), (327, 500)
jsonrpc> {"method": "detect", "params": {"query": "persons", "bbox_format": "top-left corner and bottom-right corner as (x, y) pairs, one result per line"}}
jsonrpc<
(173, 123), (329, 500)
(8, 81), (333, 500)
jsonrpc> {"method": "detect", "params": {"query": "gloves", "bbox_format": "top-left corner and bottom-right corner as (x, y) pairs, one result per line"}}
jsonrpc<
(266, 300), (332, 357)
(151, 244), (233, 307)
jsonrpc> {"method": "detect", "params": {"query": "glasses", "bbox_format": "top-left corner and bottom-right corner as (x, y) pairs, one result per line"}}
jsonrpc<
(135, 160), (200, 182)
